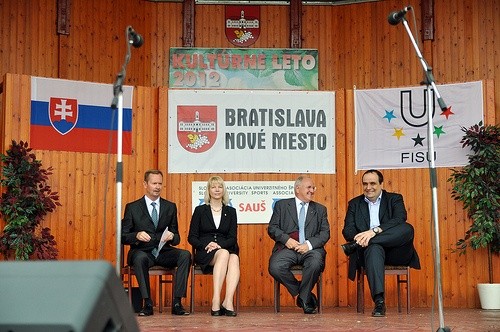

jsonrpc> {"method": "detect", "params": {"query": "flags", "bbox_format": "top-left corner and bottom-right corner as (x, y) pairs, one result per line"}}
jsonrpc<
(353, 81), (484, 174)
(31, 76), (133, 157)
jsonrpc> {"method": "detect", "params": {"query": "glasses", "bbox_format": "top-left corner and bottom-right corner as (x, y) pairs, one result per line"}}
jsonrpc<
(308, 186), (317, 191)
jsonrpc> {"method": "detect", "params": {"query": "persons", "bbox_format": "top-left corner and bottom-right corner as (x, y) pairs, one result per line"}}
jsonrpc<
(341, 170), (421, 315)
(267, 176), (330, 315)
(122, 169), (189, 317)
(188, 176), (240, 316)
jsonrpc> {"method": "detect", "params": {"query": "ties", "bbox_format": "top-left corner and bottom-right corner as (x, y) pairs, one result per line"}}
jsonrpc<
(150, 201), (158, 228)
(299, 201), (307, 244)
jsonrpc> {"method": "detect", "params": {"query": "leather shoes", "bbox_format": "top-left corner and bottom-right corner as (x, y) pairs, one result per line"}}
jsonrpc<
(211, 305), (222, 316)
(220, 304), (236, 316)
(372, 297), (387, 316)
(296, 292), (318, 313)
(341, 241), (357, 256)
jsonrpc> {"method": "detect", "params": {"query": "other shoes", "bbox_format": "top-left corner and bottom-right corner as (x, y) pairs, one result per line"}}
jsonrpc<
(138, 300), (154, 316)
(171, 301), (186, 316)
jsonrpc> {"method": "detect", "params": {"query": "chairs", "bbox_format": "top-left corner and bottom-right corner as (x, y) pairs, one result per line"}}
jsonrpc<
(356, 259), (410, 316)
(120, 219), (176, 314)
(190, 246), (240, 314)
(274, 265), (323, 313)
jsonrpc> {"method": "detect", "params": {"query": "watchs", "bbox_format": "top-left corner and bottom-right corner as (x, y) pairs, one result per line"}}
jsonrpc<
(372, 227), (379, 234)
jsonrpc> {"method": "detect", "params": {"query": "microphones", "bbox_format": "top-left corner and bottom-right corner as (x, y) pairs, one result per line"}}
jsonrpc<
(388, 6), (412, 25)
(127, 26), (145, 47)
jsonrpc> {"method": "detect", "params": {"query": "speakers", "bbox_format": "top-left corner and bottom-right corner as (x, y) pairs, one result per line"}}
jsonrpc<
(0, 260), (142, 332)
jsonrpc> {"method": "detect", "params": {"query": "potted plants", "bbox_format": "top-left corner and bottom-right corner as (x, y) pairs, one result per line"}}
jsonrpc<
(446, 118), (500, 309)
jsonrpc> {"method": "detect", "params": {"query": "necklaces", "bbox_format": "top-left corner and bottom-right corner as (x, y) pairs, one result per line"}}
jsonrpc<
(210, 206), (221, 213)
